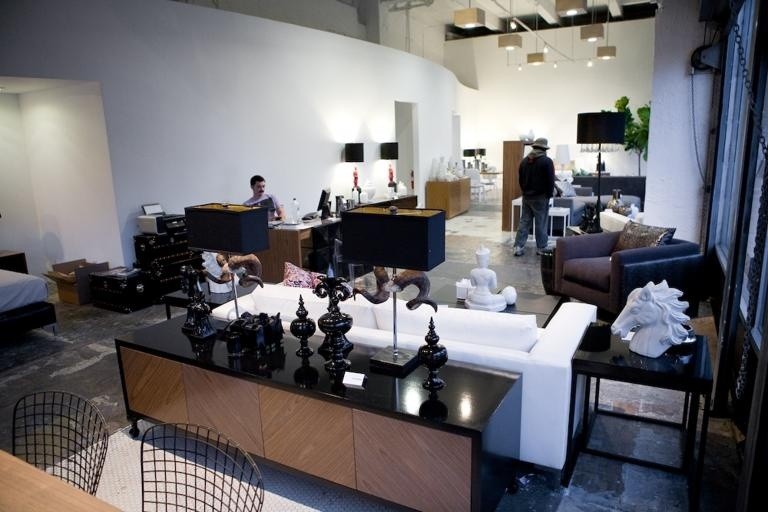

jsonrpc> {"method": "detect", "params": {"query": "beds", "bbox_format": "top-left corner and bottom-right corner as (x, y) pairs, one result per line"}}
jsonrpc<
(0, 268), (59, 338)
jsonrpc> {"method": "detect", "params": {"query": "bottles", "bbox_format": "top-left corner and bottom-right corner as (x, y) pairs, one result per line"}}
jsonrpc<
(292, 197), (300, 222)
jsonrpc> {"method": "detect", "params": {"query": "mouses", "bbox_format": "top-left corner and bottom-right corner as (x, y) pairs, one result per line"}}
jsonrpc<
(302, 217), (312, 220)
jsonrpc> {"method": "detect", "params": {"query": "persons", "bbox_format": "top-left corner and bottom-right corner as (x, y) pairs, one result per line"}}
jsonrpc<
(388, 165), (394, 181)
(242, 175), (284, 220)
(353, 167), (359, 187)
(467, 243), (505, 305)
(512, 137), (560, 255)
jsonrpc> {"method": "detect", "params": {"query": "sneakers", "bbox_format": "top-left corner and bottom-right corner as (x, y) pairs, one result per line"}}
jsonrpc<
(536, 246), (552, 256)
(513, 246), (524, 256)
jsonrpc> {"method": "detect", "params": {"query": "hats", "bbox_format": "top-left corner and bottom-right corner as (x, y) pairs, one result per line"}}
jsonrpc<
(531, 137), (550, 149)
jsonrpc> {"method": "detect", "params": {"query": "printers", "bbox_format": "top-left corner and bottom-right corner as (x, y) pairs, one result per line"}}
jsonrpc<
(136, 203), (186, 236)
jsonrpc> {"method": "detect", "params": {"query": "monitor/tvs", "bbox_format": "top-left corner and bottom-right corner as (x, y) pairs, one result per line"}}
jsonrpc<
(317, 188), (334, 220)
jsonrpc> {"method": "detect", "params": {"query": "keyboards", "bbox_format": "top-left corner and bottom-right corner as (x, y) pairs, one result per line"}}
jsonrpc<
(305, 212), (318, 219)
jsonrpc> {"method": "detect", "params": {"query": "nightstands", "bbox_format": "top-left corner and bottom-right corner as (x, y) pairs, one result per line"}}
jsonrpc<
(0, 248), (29, 274)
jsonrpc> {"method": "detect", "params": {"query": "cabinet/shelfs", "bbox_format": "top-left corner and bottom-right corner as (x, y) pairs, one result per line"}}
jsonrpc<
(113, 312), (523, 511)
(183, 174), (471, 284)
(502, 140), (534, 233)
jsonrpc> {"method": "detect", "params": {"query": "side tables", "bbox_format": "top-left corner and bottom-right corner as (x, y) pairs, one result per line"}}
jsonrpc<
(560, 322), (715, 512)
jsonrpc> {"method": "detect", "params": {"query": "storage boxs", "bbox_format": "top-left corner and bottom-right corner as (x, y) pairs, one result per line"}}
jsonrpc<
(42, 232), (206, 315)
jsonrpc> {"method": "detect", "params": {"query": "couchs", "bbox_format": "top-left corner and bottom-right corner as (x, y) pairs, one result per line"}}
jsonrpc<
(208, 285), (599, 493)
(548, 187), (641, 230)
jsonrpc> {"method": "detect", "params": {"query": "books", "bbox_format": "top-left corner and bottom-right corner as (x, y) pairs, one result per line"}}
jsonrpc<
(268, 220), (284, 226)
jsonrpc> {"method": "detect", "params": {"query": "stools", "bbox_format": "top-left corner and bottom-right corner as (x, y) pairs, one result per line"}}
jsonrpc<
(546, 206), (571, 242)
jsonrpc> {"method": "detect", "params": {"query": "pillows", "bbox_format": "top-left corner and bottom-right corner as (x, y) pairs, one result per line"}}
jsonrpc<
(554, 180), (576, 197)
(610, 218), (677, 260)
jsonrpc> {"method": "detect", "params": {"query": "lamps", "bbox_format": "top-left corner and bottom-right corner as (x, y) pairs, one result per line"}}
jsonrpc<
(380, 142), (399, 197)
(452, 0), (617, 72)
(577, 112), (626, 232)
(344, 142), (364, 205)
(463, 148), (486, 171)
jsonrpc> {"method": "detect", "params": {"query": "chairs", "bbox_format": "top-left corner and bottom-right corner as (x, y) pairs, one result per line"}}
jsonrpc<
(554, 230), (705, 324)
(0, 388), (266, 512)
